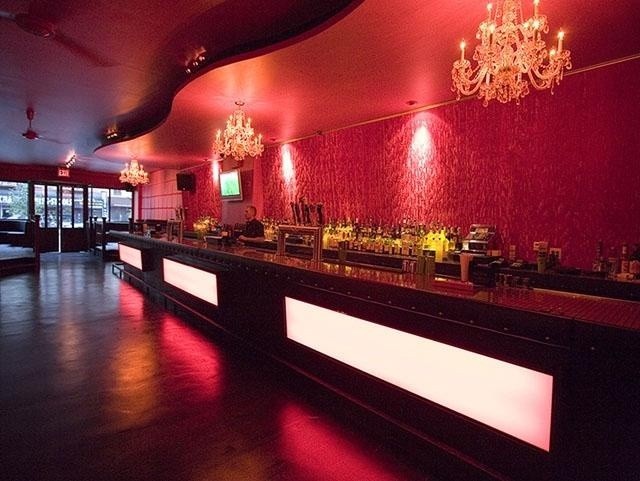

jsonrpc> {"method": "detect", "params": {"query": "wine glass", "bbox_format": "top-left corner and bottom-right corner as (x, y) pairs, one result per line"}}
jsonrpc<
(144, 226), (152, 238)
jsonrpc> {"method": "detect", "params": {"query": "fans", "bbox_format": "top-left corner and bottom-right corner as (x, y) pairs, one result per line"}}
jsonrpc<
(20, 108), (71, 146)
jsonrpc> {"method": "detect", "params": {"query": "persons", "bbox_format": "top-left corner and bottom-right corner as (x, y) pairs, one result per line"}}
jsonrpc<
(237, 206), (265, 249)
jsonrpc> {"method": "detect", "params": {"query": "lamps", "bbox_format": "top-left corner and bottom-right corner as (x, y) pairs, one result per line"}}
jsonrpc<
(449, 1), (572, 108)
(117, 159), (151, 190)
(212, 107), (266, 163)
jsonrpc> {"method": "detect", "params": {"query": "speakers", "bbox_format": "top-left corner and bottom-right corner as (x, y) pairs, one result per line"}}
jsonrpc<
(176, 173), (193, 192)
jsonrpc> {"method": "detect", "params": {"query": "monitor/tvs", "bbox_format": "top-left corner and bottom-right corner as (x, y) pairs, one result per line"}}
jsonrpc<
(219, 168), (243, 201)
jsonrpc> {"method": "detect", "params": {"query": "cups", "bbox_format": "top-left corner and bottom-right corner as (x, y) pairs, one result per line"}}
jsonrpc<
(537, 250), (546, 273)
(459, 253), (471, 281)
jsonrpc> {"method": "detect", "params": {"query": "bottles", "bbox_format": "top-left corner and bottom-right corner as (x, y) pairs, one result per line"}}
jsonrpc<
(192, 213), (247, 239)
(402, 255), (435, 275)
(591, 238), (640, 279)
(261, 216), (461, 260)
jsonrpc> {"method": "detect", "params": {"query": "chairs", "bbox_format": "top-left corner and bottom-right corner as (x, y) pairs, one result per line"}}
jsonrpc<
(88, 215), (147, 264)
(1, 215), (40, 279)
